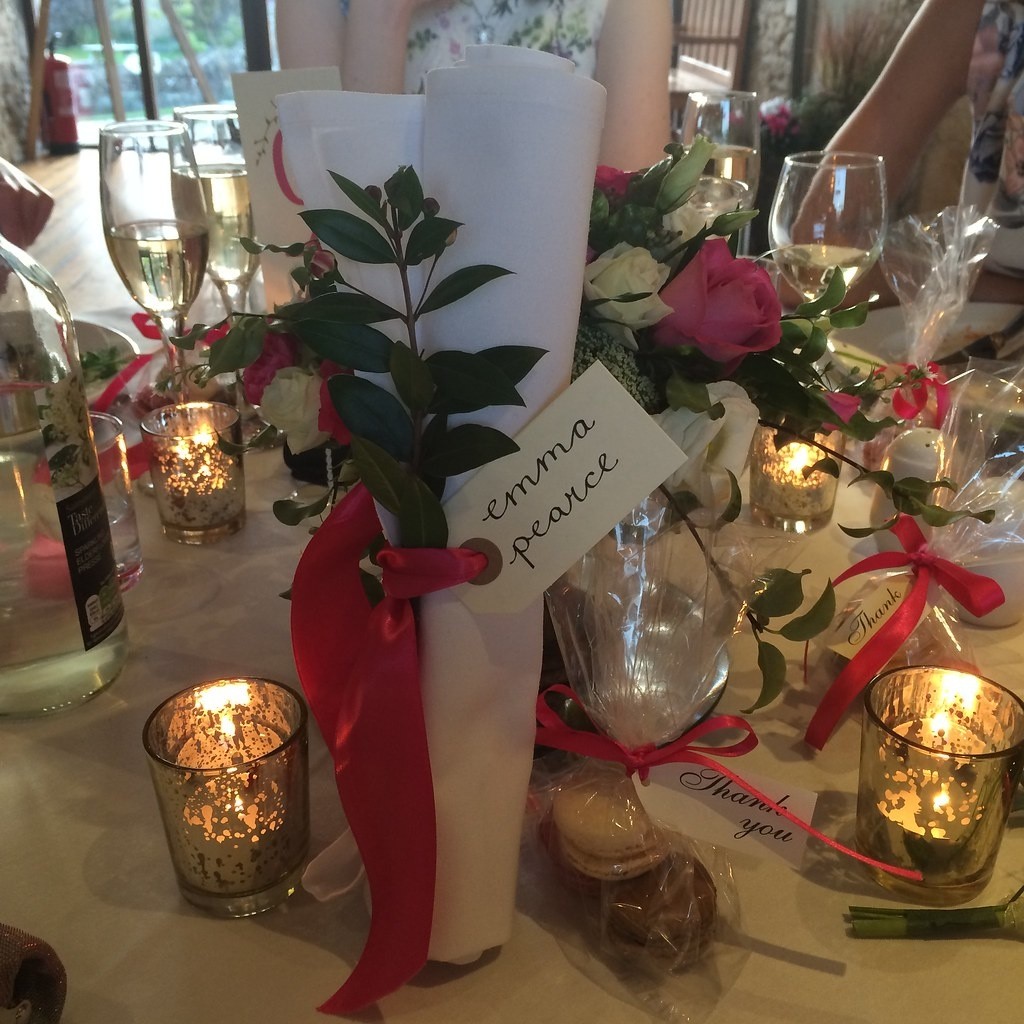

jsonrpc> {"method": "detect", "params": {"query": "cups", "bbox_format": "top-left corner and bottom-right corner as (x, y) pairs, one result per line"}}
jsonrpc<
(749, 418), (848, 535)
(89, 410), (144, 590)
(852, 666), (1024, 906)
(142, 675), (315, 917)
(139, 401), (246, 542)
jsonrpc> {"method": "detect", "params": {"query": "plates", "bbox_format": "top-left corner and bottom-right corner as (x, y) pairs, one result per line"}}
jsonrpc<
(0, 316), (138, 424)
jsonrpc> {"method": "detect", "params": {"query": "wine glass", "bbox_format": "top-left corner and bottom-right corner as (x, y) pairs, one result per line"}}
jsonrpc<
(171, 103), (261, 333)
(98, 118), (213, 401)
(769, 151), (890, 346)
(677, 87), (762, 239)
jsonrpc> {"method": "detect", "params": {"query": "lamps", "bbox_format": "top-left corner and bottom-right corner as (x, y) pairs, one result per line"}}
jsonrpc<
(852, 664), (1024, 907)
(142, 675), (311, 919)
(750, 417), (846, 532)
(141, 400), (247, 548)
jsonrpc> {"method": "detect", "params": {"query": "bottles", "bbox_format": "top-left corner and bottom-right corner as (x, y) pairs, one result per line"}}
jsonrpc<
(0, 231), (128, 720)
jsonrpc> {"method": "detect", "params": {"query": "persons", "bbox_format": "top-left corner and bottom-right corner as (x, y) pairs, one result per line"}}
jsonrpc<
(782, 0), (1024, 303)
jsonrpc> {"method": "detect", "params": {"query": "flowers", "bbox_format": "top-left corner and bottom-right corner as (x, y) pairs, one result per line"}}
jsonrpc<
(182, 129), (996, 751)
(729, 96), (801, 143)
